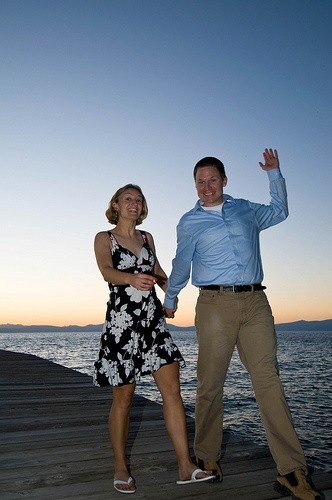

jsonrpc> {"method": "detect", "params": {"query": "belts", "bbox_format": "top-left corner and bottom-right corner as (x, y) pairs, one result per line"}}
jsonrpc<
(199, 284), (268, 292)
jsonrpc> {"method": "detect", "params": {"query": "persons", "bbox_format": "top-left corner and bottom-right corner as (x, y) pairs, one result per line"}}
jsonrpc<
(93, 184), (215, 493)
(163, 148), (323, 500)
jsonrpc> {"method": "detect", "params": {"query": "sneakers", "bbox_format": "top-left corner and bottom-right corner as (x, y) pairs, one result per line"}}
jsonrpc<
(196, 455), (222, 482)
(275, 469), (323, 500)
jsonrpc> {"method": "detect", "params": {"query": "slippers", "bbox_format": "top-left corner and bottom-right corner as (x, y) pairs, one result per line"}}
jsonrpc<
(113, 477), (137, 493)
(176, 468), (215, 485)
